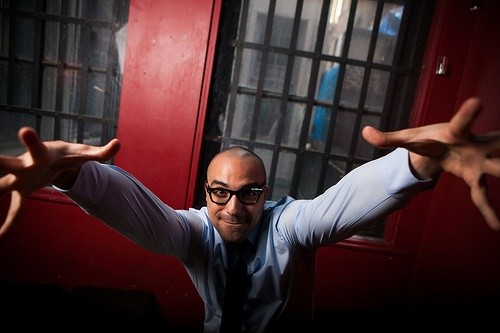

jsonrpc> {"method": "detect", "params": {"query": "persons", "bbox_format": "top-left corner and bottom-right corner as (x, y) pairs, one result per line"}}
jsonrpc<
(0, 96), (500, 333)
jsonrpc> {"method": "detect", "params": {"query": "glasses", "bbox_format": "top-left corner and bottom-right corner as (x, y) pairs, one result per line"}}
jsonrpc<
(204, 183), (267, 206)
